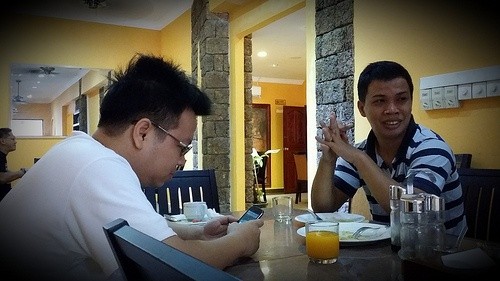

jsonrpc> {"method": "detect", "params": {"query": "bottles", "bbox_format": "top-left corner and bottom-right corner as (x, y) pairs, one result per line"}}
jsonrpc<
(387, 184), (447, 267)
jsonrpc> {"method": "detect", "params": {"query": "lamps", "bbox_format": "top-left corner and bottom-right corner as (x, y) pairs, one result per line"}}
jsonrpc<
(10, 80), (27, 104)
(38, 67), (59, 78)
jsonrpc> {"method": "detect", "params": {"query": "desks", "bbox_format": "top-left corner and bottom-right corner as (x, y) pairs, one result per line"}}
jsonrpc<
(165, 203), (500, 281)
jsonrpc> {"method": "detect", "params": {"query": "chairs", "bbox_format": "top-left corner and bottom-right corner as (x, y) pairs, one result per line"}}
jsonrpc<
(141, 169), (221, 218)
(292, 150), (308, 204)
(456, 168), (500, 245)
(252, 150), (267, 203)
(454, 153), (473, 169)
(102, 218), (241, 281)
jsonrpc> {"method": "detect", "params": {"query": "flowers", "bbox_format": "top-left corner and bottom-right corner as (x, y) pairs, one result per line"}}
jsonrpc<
(250, 147), (283, 197)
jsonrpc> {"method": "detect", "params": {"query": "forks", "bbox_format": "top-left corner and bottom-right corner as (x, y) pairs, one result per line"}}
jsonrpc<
(353, 225), (382, 237)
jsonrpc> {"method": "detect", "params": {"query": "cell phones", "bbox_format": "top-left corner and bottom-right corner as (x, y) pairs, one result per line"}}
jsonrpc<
(236, 205), (264, 224)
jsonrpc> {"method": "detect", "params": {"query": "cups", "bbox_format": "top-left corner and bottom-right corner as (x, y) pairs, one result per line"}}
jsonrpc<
(183, 201), (207, 223)
(272, 195), (294, 224)
(304, 220), (339, 265)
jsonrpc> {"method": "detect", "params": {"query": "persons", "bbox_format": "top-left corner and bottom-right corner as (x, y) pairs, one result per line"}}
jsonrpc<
(0, 52), (264, 281)
(0, 127), (31, 202)
(311, 61), (469, 238)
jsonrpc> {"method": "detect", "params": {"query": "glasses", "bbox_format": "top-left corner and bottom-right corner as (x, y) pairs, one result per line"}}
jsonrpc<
(131, 113), (194, 156)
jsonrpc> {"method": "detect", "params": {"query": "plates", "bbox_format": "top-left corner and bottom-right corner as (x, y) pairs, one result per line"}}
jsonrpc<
(294, 212), (366, 222)
(296, 221), (391, 242)
